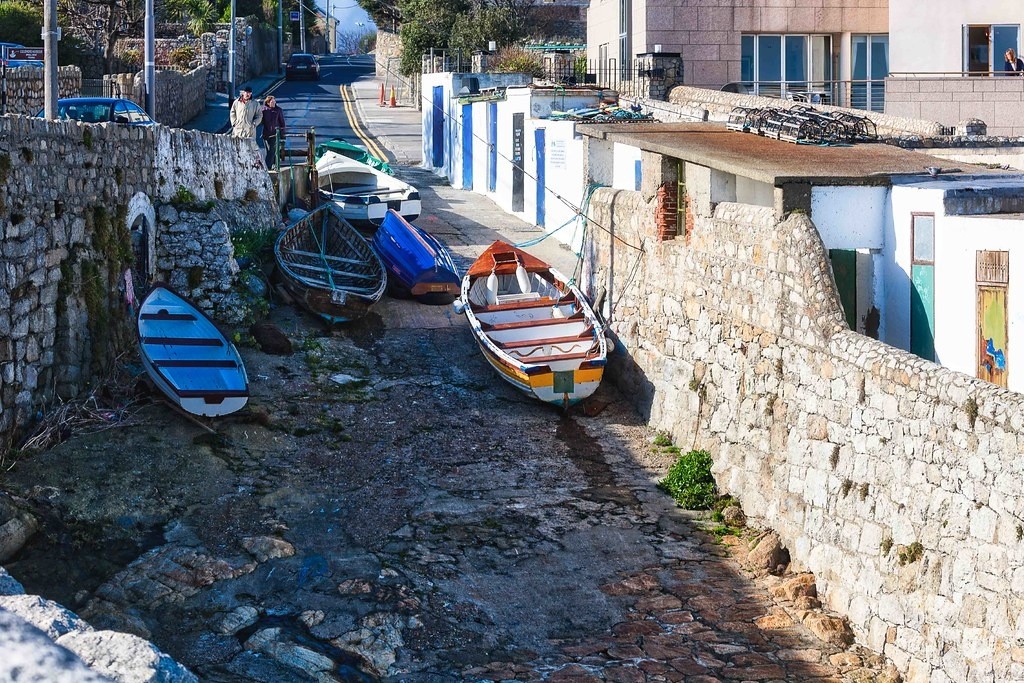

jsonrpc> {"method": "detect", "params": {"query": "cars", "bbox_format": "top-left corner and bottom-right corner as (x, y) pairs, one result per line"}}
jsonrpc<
(33, 97), (163, 128)
(0, 43), (44, 67)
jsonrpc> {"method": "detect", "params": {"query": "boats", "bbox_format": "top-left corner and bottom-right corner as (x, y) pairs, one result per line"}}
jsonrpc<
(308, 151), (422, 229)
(272, 202), (387, 324)
(135, 283), (250, 418)
(312, 139), (382, 175)
(370, 208), (462, 306)
(460, 240), (608, 409)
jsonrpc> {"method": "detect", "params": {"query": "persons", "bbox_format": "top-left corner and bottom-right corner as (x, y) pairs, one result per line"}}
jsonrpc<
(1004, 48), (1024, 76)
(65, 106), (78, 120)
(230, 86), (263, 138)
(261, 95), (286, 170)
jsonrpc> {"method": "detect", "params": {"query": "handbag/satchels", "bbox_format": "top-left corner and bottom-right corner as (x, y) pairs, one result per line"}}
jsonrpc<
(280, 147), (285, 161)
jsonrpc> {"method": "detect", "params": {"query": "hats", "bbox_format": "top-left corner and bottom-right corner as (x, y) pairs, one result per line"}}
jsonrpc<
(244, 87), (252, 91)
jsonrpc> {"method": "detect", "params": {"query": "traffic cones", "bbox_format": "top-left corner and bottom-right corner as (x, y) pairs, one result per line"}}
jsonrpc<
(388, 84), (398, 108)
(377, 83), (386, 104)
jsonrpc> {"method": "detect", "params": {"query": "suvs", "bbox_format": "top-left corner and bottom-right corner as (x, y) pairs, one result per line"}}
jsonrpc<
(286, 53), (320, 80)
